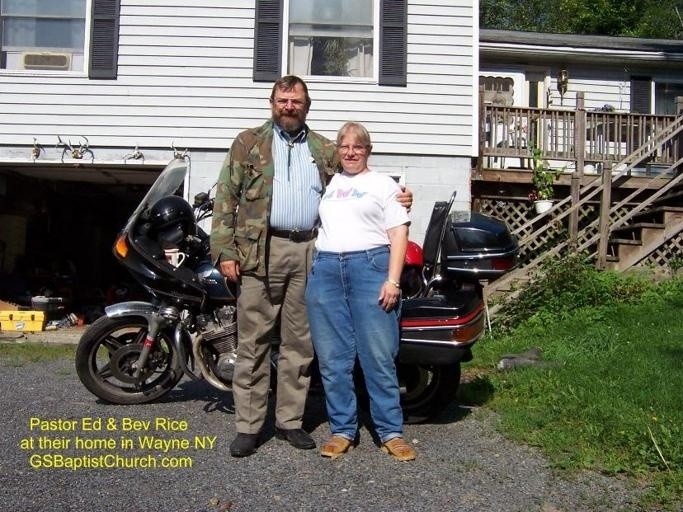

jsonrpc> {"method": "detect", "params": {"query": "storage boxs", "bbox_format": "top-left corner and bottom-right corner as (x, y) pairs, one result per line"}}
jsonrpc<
(0, 311), (45, 331)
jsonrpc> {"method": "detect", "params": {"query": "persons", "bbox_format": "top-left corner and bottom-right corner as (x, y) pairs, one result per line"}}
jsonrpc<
(307, 114), (417, 462)
(206, 76), (414, 456)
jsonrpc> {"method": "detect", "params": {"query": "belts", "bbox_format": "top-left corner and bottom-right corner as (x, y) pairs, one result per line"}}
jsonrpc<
(265, 228), (318, 242)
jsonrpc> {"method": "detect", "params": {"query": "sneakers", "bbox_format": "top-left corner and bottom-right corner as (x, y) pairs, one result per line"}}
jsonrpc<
(319, 434), (354, 456)
(229, 432), (267, 458)
(273, 427), (316, 450)
(380, 437), (420, 462)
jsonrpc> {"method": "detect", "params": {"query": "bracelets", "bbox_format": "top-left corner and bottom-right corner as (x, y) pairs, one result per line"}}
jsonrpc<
(388, 278), (401, 289)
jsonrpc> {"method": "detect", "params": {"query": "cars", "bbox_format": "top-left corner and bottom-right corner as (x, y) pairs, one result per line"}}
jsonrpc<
(0, 263), (72, 317)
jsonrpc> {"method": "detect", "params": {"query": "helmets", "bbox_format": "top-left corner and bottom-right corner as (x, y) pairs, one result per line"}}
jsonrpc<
(150, 196), (195, 239)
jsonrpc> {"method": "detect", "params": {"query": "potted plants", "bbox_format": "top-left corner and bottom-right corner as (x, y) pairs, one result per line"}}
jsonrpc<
(523, 134), (567, 214)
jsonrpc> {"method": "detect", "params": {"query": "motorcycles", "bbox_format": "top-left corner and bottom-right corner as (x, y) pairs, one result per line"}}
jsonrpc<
(73, 155), (518, 426)
